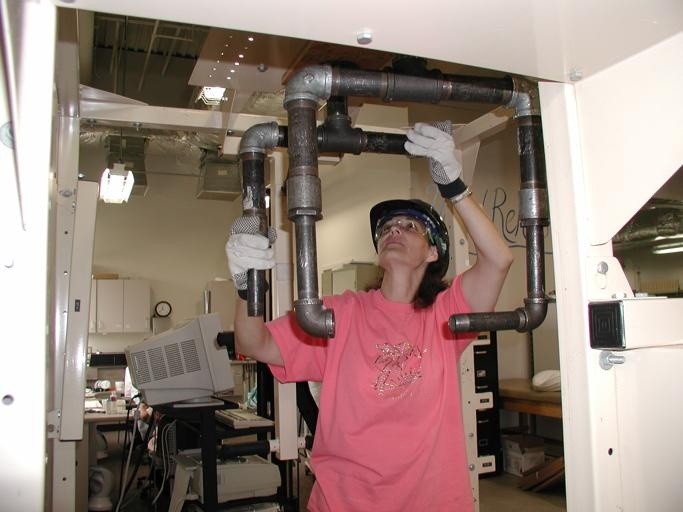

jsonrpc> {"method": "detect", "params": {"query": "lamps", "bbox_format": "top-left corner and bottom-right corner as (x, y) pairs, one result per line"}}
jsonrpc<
(99, 164), (135, 204)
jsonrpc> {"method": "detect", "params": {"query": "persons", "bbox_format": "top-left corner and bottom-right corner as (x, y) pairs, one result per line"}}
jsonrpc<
(224, 119), (515, 511)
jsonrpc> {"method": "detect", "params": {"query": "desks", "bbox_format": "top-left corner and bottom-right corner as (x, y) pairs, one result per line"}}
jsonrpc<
(497, 378), (562, 419)
(153, 398), (288, 512)
(76, 399), (140, 512)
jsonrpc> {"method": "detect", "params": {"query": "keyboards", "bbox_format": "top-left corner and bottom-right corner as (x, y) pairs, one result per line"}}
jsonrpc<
(214, 407), (275, 430)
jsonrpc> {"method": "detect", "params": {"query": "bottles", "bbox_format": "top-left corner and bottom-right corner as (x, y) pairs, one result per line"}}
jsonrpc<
(107, 397), (118, 416)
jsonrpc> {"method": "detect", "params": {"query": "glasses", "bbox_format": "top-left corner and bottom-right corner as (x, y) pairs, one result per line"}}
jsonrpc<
(375, 217), (435, 244)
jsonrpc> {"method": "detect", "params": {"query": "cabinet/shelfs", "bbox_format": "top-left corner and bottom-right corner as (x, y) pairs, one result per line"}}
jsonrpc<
(89, 277), (152, 335)
(320, 266), (383, 297)
(204, 279), (235, 330)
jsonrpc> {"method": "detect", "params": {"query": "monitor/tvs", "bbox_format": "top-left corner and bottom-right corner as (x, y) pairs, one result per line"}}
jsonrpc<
(124, 312), (235, 408)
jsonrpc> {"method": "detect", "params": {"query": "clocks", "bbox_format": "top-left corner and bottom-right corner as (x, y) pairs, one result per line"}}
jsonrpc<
(155, 301), (172, 317)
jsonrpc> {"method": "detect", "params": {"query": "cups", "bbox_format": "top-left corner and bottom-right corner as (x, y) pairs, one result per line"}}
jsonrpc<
(114, 381), (125, 394)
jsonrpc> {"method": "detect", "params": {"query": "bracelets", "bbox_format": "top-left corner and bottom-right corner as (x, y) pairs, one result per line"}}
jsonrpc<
(443, 184), (473, 206)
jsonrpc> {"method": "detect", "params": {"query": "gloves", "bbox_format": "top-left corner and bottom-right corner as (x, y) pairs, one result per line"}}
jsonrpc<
(225, 218), (278, 299)
(405, 122), (467, 198)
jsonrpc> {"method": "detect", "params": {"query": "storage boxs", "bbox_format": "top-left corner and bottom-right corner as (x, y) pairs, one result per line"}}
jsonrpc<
(500, 434), (554, 477)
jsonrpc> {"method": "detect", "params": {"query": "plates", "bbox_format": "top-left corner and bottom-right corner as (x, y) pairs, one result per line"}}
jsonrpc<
(93, 380), (111, 389)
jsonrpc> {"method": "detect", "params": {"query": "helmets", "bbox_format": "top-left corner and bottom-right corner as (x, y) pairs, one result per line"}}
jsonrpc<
(370, 199), (450, 255)
